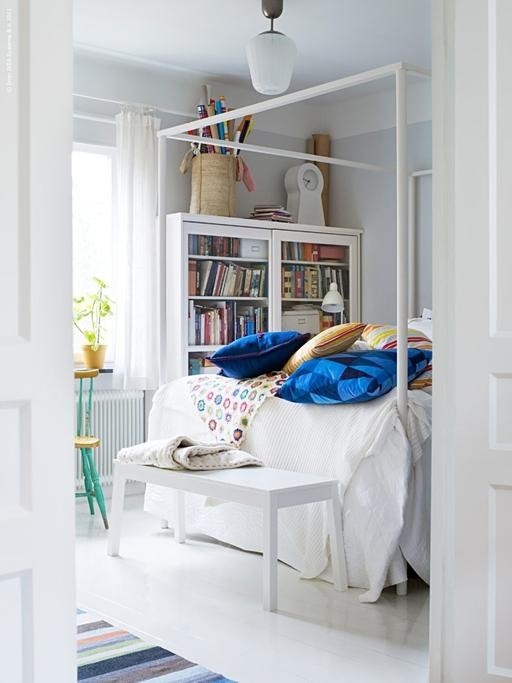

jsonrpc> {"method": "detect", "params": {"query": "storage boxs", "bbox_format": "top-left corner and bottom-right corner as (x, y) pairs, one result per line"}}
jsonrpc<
(282, 309), (320, 336)
(239, 238), (268, 259)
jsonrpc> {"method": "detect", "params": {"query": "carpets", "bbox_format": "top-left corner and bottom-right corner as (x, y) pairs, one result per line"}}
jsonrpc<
(78, 608), (239, 683)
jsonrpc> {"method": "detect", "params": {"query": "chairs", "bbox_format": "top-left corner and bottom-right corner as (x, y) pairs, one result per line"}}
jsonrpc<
(73, 369), (109, 529)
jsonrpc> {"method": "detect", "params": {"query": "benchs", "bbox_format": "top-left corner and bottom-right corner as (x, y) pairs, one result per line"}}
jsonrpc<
(108, 460), (348, 612)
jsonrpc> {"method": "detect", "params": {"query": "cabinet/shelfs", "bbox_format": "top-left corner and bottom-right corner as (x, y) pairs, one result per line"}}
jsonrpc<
(165, 212), (363, 381)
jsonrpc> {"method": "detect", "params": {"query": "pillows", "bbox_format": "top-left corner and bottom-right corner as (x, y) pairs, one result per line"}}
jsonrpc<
(273, 348), (432, 405)
(281, 322), (366, 376)
(362, 323), (432, 390)
(205, 331), (311, 379)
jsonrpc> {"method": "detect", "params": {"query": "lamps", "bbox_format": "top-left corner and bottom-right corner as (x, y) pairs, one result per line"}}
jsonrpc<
(246, 0), (297, 95)
(321, 282), (345, 325)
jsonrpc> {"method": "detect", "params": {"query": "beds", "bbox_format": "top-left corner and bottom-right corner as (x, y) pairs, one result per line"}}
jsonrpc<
(147, 61), (432, 597)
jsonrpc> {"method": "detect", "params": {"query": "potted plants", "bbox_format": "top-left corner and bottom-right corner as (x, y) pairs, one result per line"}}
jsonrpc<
(73, 277), (112, 369)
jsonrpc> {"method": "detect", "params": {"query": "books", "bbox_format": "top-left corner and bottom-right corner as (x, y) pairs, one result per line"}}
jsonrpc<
(282, 265), (349, 299)
(282, 242), (319, 261)
(248, 204), (292, 223)
(189, 259), (268, 297)
(319, 308), (348, 334)
(188, 300), (268, 345)
(188, 234), (240, 257)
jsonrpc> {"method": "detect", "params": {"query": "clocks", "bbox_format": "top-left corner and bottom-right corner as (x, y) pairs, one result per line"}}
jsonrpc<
(283, 162), (325, 227)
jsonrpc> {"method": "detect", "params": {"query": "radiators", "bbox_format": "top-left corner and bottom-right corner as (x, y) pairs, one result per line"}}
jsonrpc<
(74, 389), (144, 490)
